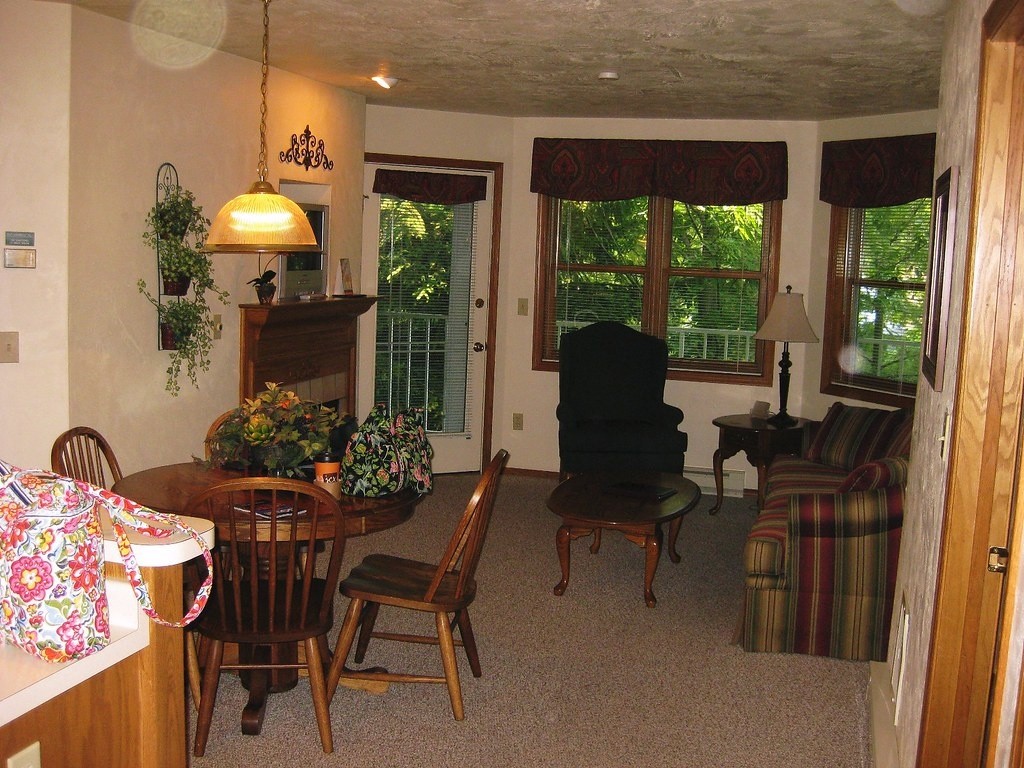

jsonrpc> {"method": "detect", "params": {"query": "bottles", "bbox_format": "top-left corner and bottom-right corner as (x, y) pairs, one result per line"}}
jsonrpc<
(297, 273), (310, 295)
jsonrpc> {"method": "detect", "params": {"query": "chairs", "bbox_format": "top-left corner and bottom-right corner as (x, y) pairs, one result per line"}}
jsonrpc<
(555, 320), (688, 481)
(50, 425), (124, 489)
(186, 477), (347, 753)
(203, 409), (244, 461)
(326, 447), (511, 720)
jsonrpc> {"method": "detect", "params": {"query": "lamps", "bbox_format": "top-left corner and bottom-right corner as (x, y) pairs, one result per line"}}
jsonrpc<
(375, 78), (399, 89)
(755, 285), (819, 427)
(201, 0), (322, 254)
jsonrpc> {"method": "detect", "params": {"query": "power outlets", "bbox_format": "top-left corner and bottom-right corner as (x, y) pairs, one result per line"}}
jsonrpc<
(8, 741), (42, 768)
(513, 413), (523, 430)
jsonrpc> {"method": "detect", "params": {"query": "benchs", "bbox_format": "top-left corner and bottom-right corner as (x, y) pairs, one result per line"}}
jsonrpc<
(739, 402), (916, 662)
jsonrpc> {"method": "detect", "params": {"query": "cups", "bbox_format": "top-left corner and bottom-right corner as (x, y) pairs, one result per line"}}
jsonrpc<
(315, 452), (342, 503)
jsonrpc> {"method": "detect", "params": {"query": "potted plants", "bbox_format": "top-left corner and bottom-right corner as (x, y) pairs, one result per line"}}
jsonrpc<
(135, 184), (230, 398)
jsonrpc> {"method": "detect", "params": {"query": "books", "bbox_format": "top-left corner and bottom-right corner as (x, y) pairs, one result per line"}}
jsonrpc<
(227, 497), (307, 521)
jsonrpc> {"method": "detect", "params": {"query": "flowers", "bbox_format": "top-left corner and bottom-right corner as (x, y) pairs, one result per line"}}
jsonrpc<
(187, 378), (349, 475)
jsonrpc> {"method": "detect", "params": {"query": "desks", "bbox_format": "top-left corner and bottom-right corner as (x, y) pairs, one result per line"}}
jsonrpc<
(546, 472), (702, 607)
(118, 461), (419, 693)
(708, 413), (815, 516)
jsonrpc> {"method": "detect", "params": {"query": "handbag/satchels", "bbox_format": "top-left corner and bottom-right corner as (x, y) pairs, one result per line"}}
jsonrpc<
(0, 460), (214, 664)
(338, 404), (435, 498)
